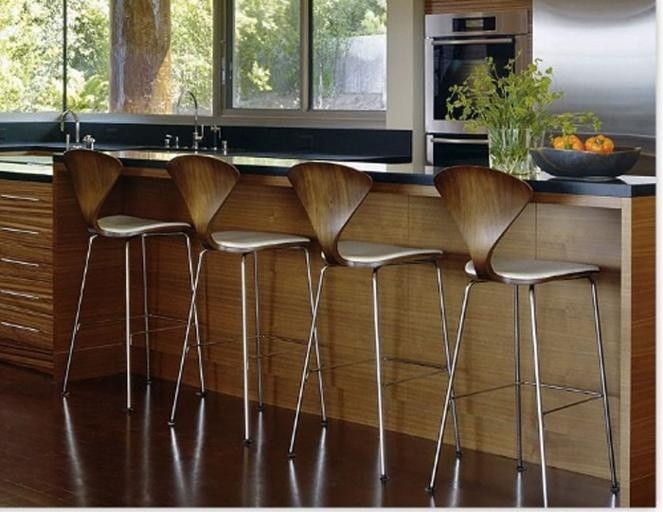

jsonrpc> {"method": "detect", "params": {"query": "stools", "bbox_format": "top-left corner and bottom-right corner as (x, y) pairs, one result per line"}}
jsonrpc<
(166, 154), (327, 445)
(284, 160), (463, 484)
(427, 169), (620, 507)
(63, 148), (206, 410)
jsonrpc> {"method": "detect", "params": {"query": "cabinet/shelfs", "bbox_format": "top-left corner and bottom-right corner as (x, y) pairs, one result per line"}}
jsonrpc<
(0, 176), (130, 384)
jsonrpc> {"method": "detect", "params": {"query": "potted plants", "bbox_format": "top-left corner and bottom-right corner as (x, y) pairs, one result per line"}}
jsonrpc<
(445, 55), (604, 179)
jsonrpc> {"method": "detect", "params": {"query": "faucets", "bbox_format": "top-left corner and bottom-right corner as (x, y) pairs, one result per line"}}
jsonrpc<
(177, 89), (205, 148)
(59, 108), (81, 143)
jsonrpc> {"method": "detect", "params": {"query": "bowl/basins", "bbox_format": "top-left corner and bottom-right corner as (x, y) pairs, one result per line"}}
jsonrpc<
(529, 145), (642, 180)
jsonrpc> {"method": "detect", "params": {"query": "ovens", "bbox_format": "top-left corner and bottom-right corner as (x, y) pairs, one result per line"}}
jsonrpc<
(425, 9), (528, 169)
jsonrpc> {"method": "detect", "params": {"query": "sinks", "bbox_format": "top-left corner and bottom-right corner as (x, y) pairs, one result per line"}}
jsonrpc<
(0, 143), (72, 164)
(134, 147), (204, 153)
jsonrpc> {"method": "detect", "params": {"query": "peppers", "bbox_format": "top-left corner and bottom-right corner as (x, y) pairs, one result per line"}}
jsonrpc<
(584, 135), (615, 153)
(553, 134), (584, 151)
